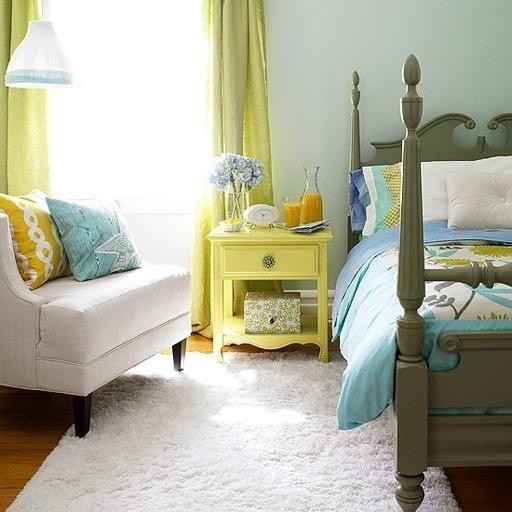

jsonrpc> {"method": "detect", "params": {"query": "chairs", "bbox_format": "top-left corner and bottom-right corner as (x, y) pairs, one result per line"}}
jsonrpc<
(0, 215), (194, 437)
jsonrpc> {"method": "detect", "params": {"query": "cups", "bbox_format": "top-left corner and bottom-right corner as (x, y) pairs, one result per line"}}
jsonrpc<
(281, 194), (302, 229)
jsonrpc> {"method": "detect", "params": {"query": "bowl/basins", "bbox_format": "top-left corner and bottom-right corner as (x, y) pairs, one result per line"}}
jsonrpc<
(218, 221), (242, 232)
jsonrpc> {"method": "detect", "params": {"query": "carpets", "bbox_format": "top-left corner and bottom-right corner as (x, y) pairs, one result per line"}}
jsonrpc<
(3, 349), (462, 512)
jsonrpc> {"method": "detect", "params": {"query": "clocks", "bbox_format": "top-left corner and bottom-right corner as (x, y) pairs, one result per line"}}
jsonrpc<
(244, 204), (280, 232)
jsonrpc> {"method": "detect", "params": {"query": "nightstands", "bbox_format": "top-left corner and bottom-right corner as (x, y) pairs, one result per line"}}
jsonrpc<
(205, 224), (334, 362)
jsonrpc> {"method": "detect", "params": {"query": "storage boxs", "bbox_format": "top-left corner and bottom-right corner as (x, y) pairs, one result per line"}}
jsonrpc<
(244, 291), (301, 335)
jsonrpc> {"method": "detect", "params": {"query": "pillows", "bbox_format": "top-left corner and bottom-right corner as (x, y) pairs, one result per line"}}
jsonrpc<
(362, 155), (512, 231)
(0, 190), (69, 290)
(46, 198), (141, 281)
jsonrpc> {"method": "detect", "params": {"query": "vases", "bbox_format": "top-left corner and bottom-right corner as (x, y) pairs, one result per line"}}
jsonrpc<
(226, 188), (249, 226)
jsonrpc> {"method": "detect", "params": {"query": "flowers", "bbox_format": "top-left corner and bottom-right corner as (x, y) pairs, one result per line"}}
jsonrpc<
(211, 153), (265, 217)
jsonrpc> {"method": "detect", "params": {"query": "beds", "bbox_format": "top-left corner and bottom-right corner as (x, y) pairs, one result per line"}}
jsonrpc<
(337, 52), (512, 512)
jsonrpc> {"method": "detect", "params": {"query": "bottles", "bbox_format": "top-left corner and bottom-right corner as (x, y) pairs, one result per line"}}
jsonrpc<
(301, 165), (323, 225)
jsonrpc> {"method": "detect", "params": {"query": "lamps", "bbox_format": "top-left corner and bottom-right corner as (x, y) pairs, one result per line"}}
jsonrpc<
(5, 0), (71, 89)
